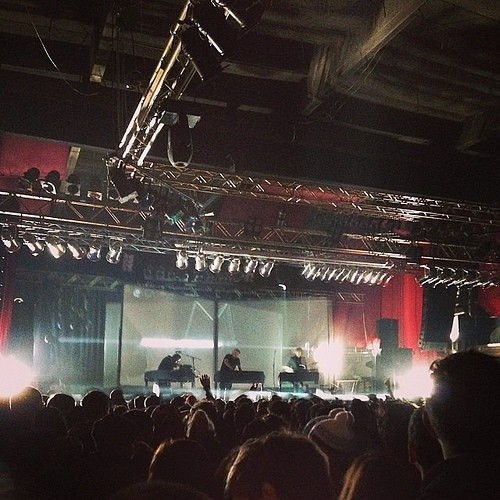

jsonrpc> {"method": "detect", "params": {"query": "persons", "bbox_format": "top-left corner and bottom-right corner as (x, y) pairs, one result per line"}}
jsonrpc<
(0, 351), (500, 500)
(286, 348), (307, 394)
(220, 348), (243, 401)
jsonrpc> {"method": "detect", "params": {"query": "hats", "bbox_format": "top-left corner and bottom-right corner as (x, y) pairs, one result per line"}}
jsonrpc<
(303, 407), (345, 437)
(308, 411), (358, 452)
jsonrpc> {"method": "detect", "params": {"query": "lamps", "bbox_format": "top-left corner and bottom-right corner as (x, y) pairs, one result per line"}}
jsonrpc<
(1, 233), (122, 264)
(16, 153), (157, 206)
(303, 264), (393, 289)
(176, 249), (276, 279)
(415, 266), (499, 291)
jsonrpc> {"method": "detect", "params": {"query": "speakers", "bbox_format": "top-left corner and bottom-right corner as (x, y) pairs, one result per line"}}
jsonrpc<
(418, 267), (480, 351)
(372, 318), (413, 392)
